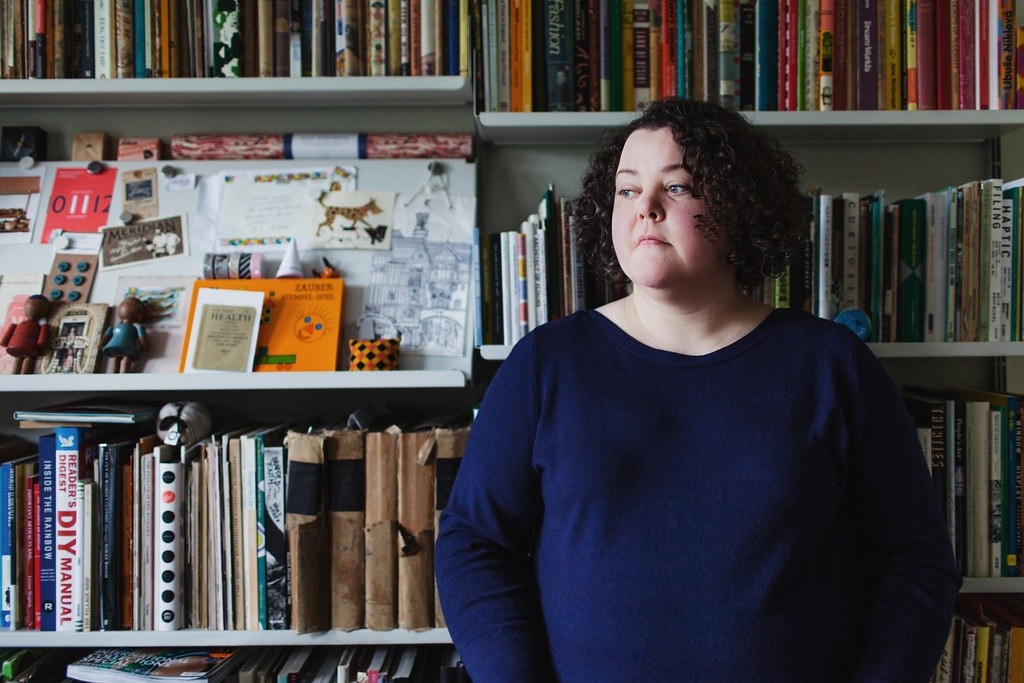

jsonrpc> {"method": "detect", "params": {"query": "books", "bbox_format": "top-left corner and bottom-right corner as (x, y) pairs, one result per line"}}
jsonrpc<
(907, 389), (1024, 683)
(469, 177), (1024, 350)
(0, 0), (1024, 111)
(0, 394), (471, 683)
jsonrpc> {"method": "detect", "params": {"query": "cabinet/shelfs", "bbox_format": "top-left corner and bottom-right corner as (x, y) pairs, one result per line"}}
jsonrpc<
(0, 0), (1024, 683)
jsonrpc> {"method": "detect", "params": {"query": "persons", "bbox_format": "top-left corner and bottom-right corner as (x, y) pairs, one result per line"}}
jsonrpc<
(0, 294), (52, 374)
(430, 94), (963, 683)
(99, 298), (148, 374)
